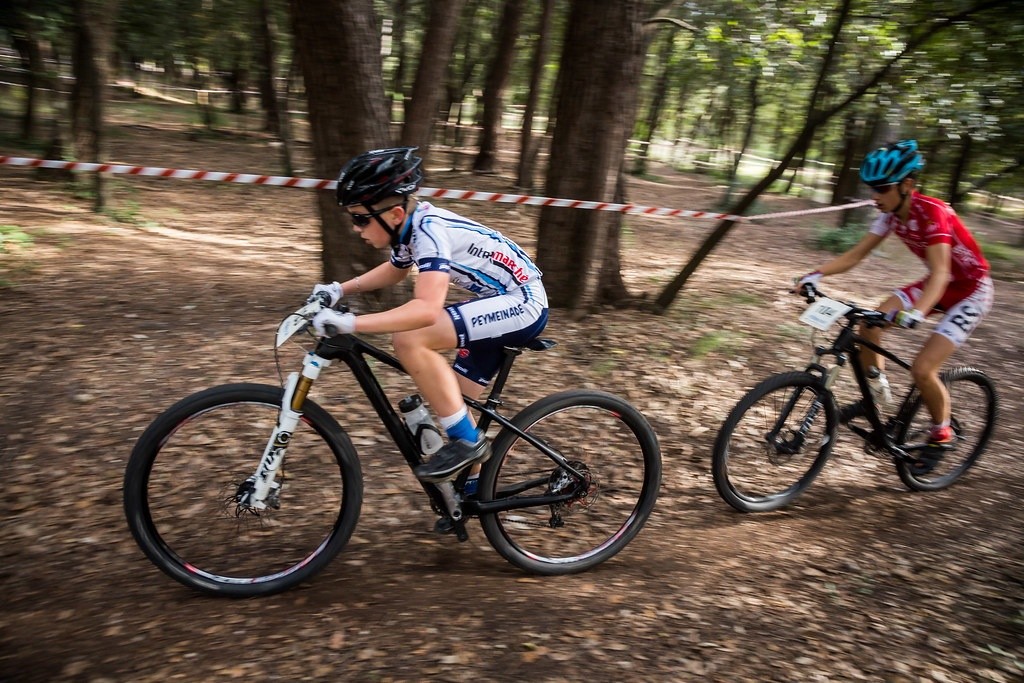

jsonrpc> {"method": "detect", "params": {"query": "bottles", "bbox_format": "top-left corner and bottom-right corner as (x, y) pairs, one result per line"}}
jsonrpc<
(398, 394), (444, 454)
(866, 365), (892, 405)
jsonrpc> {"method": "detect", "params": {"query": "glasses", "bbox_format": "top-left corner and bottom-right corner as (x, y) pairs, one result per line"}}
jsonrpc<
(346, 199), (410, 227)
(871, 185), (900, 194)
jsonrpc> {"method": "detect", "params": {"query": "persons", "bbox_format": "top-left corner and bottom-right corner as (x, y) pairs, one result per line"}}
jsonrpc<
(793, 140), (994, 455)
(309, 148), (550, 476)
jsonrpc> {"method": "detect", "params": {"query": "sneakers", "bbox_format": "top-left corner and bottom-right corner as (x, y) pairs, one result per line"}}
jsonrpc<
(909, 427), (957, 447)
(434, 514), (474, 535)
(414, 429), (495, 482)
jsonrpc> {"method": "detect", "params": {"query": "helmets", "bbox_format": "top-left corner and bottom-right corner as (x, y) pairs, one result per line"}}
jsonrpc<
(336, 144), (425, 208)
(859, 139), (927, 187)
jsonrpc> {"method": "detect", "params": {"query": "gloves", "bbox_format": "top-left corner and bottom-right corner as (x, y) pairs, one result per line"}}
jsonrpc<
(312, 281), (345, 308)
(312, 308), (356, 336)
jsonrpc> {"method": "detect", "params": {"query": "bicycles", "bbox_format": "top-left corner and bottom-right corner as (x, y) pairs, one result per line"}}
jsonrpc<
(706, 277), (998, 514)
(121, 286), (667, 598)
